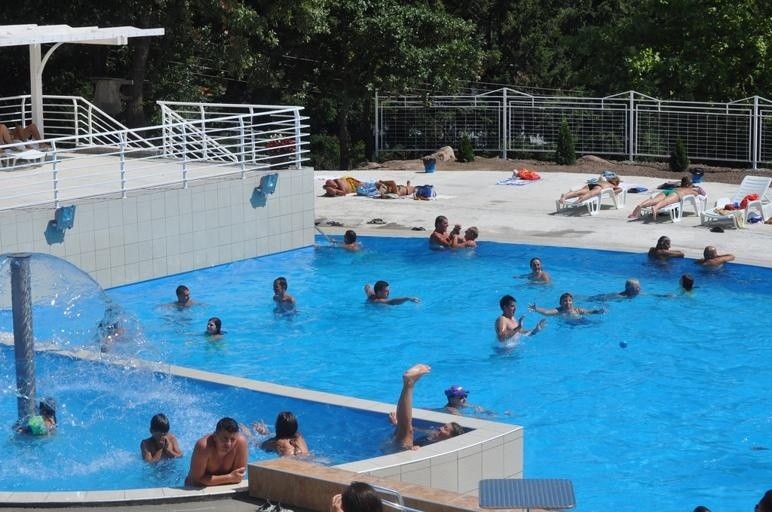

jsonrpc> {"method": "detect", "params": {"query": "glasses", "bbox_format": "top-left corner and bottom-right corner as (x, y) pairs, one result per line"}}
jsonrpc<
(454, 394), (467, 400)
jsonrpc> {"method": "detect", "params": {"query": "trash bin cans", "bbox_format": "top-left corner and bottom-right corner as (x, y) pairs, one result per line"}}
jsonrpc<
(423, 158), (436, 173)
(692, 167), (704, 182)
(342, 146), (358, 170)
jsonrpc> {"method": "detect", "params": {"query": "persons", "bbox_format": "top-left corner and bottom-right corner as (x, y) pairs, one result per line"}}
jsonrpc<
(649, 236), (735, 292)
(184, 417), (248, 487)
(323, 177), (416, 198)
(0, 124), (20, 153)
(9, 122), (50, 150)
(96, 306), (126, 352)
(272, 277), (297, 318)
(15, 397), (59, 435)
(388, 364), (462, 452)
(433, 385), (485, 418)
(170, 286), (205, 313)
(344, 230), (357, 246)
(237, 410), (310, 460)
(559, 176), (621, 204)
(182, 316), (231, 353)
(692, 489), (772, 512)
(430, 215), (479, 247)
(140, 413), (183, 465)
(633, 176), (700, 219)
(366, 280), (408, 305)
(495, 257), (640, 341)
(329, 481), (384, 512)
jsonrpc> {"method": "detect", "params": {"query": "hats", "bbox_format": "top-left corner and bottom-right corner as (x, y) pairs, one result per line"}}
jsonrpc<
(444, 385), (465, 398)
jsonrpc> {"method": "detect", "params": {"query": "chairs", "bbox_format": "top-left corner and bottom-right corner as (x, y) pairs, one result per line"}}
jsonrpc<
(699, 175), (772, 229)
(0, 137), (76, 172)
(641, 187), (708, 223)
(555, 183), (632, 216)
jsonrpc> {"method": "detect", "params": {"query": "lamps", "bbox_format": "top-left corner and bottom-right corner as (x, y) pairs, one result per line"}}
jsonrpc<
(49, 204), (76, 232)
(255, 172), (279, 195)
